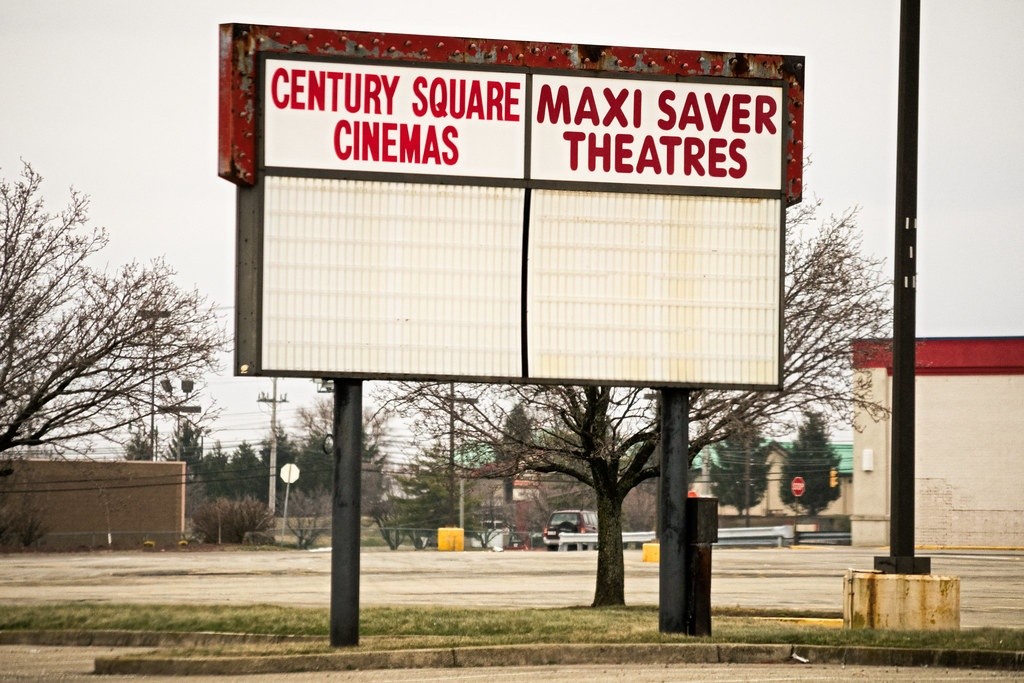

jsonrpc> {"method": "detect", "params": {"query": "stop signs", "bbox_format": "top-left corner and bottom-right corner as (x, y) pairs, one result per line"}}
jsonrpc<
(792, 476), (805, 499)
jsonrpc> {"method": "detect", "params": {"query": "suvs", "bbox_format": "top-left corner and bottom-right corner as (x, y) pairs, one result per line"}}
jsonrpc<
(546, 508), (601, 550)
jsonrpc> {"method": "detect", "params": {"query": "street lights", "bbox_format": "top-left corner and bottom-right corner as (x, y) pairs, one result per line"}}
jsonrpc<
(157, 379), (201, 465)
